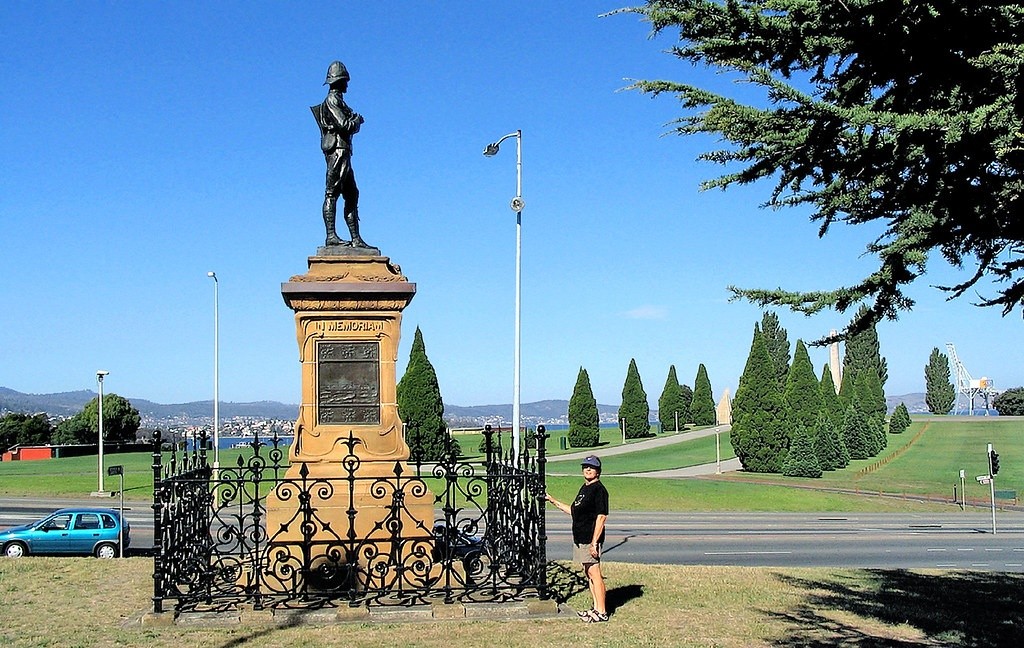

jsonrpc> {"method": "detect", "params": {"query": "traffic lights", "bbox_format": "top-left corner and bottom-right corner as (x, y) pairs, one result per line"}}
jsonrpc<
(987, 451), (1001, 478)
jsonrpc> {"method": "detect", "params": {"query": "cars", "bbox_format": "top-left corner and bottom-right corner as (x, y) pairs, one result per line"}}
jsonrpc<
(0, 509), (130, 560)
(434, 527), (489, 560)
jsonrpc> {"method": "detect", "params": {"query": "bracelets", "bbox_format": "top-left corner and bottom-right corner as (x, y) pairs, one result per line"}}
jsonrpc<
(590, 543), (597, 547)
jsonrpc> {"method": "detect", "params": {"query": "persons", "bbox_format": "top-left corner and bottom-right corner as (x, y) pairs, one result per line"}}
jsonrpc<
(545, 455), (611, 623)
(309, 61), (378, 249)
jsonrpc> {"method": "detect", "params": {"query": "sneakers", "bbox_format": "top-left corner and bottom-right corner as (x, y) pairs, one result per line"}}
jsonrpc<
(580, 610), (609, 623)
(577, 607), (600, 617)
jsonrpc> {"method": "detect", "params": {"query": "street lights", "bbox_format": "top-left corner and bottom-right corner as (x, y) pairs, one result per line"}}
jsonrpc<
(484, 129), (524, 513)
(208, 270), (220, 508)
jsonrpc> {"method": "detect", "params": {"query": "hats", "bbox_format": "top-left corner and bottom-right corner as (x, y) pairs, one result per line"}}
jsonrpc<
(581, 457), (600, 466)
